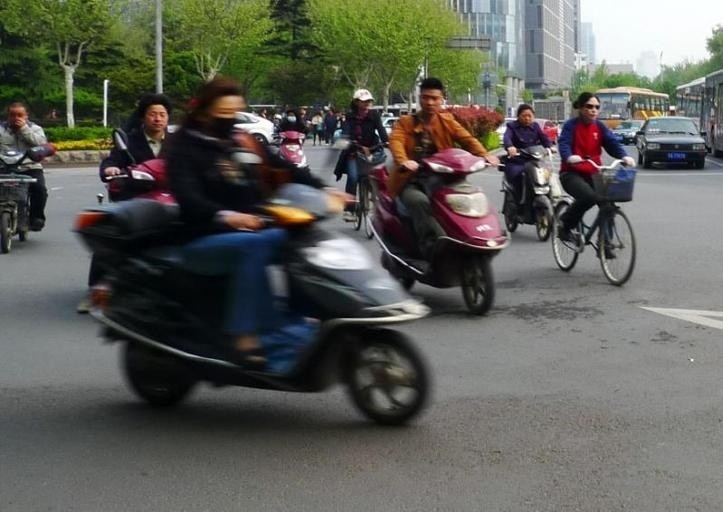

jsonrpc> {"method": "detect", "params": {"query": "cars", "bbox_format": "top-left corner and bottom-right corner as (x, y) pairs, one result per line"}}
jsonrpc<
(333, 116), (398, 141)
(610, 123), (640, 145)
(165, 112), (275, 148)
(494, 118), (517, 143)
(636, 117), (708, 169)
(534, 119), (557, 145)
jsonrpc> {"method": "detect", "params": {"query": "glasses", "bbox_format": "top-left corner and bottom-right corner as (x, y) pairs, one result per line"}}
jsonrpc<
(584, 103), (600, 110)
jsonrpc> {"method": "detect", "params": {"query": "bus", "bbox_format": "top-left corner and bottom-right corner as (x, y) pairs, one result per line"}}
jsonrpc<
(676, 69), (722, 158)
(594, 86), (670, 130)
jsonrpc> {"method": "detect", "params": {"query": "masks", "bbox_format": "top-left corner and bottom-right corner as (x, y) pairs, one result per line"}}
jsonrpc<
(288, 116), (296, 122)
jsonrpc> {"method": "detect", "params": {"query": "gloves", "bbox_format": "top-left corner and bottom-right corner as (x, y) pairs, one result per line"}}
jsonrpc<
(568, 155), (583, 164)
(623, 156), (634, 167)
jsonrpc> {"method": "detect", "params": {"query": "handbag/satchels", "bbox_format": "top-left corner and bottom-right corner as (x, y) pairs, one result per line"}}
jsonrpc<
(357, 150), (386, 166)
(548, 172), (560, 201)
(416, 129), (438, 177)
(608, 168), (636, 197)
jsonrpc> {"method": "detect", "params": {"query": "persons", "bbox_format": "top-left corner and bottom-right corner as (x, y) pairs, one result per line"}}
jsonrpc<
(333, 89), (389, 223)
(0, 102), (48, 231)
(503, 104), (554, 219)
(558, 92), (636, 259)
(77, 94), (171, 313)
(385, 78), (500, 259)
(166, 76), (357, 372)
(251, 102), (349, 146)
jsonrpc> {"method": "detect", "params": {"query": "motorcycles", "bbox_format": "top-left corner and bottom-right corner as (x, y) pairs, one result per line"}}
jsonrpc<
(496, 144), (557, 240)
(68, 182), (431, 423)
(0, 143), (55, 253)
(272, 130), (307, 169)
(366, 149), (512, 316)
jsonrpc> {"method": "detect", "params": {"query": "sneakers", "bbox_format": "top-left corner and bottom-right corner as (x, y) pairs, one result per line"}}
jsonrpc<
(558, 217), (570, 241)
(29, 218), (44, 227)
(77, 295), (91, 315)
(343, 212), (356, 222)
(598, 248), (616, 258)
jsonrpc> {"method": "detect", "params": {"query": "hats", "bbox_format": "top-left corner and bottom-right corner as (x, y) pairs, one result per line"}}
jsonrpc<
(353, 89), (375, 101)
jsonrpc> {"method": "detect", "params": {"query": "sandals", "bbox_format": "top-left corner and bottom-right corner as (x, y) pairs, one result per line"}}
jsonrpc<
(234, 339), (268, 372)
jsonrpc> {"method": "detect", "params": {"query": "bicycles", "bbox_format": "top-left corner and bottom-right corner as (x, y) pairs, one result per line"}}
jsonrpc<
(550, 154), (636, 286)
(344, 142), (390, 240)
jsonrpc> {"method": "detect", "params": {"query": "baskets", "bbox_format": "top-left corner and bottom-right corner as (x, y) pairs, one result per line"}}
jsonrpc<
(0, 174), (32, 201)
(591, 171), (634, 202)
